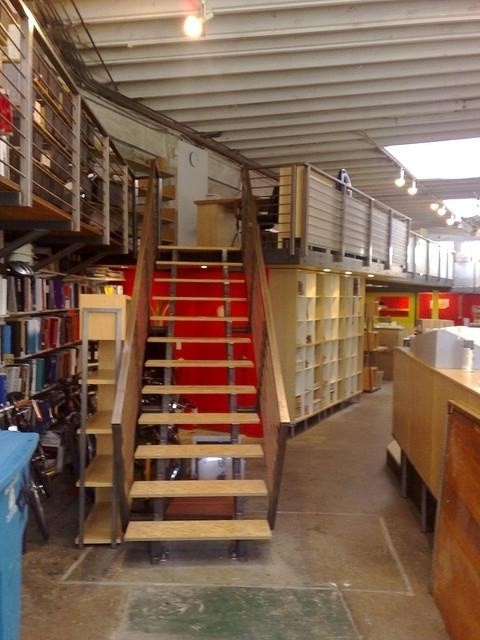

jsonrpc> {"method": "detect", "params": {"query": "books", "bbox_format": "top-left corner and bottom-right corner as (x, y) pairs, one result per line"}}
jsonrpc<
(1, 275), (80, 412)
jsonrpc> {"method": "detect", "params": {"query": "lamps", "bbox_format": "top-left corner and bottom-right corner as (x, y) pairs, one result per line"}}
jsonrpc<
(182, 1), (213, 39)
(393, 168), (455, 226)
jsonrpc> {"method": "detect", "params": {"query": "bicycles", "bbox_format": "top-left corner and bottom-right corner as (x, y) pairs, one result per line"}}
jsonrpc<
(0, 363), (201, 544)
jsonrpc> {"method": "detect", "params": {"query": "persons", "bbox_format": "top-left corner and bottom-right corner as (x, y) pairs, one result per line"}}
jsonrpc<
(337, 169), (353, 196)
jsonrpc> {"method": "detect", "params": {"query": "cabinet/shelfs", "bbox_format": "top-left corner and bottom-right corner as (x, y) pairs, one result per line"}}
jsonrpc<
(0, 277), (126, 476)
(265, 265), (368, 436)
